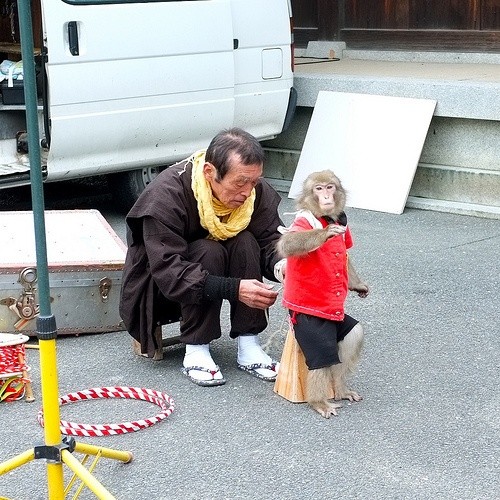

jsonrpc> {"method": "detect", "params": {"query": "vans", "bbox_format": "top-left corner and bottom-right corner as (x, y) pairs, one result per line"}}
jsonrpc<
(1, 0), (296, 208)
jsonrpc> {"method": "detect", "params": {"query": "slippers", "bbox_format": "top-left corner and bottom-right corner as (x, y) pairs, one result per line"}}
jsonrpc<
(236, 359), (283, 382)
(179, 362), (226, 386)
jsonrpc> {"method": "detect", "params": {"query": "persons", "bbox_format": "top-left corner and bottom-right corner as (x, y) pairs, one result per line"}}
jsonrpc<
(119, 127), (291, 388)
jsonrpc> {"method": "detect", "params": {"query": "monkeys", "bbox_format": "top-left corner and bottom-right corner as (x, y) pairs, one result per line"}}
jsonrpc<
(275, 170), (369, 418)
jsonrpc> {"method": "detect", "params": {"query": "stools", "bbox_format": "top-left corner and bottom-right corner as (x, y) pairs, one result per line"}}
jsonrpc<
(135, 310), (184, 361)
(0, 332), (35, 403)
